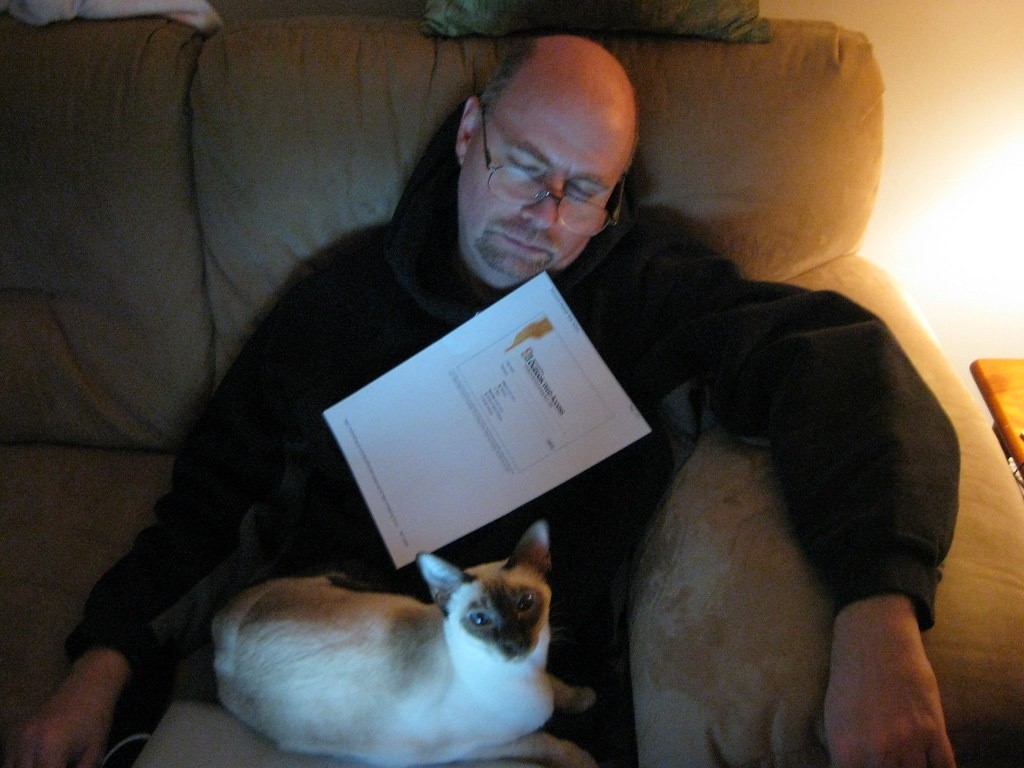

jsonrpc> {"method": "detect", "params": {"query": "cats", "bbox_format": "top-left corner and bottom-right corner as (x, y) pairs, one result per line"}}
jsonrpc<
(209, 520), (599, 768)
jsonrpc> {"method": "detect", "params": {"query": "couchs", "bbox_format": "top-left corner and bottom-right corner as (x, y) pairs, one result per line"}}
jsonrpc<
(0, 0), (1024, 768)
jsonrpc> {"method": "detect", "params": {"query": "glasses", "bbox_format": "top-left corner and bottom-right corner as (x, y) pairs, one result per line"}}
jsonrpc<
(480, 106), (627, 236)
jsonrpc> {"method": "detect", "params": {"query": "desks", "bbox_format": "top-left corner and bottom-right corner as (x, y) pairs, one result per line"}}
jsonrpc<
(971, 357), (1024, 480)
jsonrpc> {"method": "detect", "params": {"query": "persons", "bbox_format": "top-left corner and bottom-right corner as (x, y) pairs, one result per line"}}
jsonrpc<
(0, 31), (963, 768)
